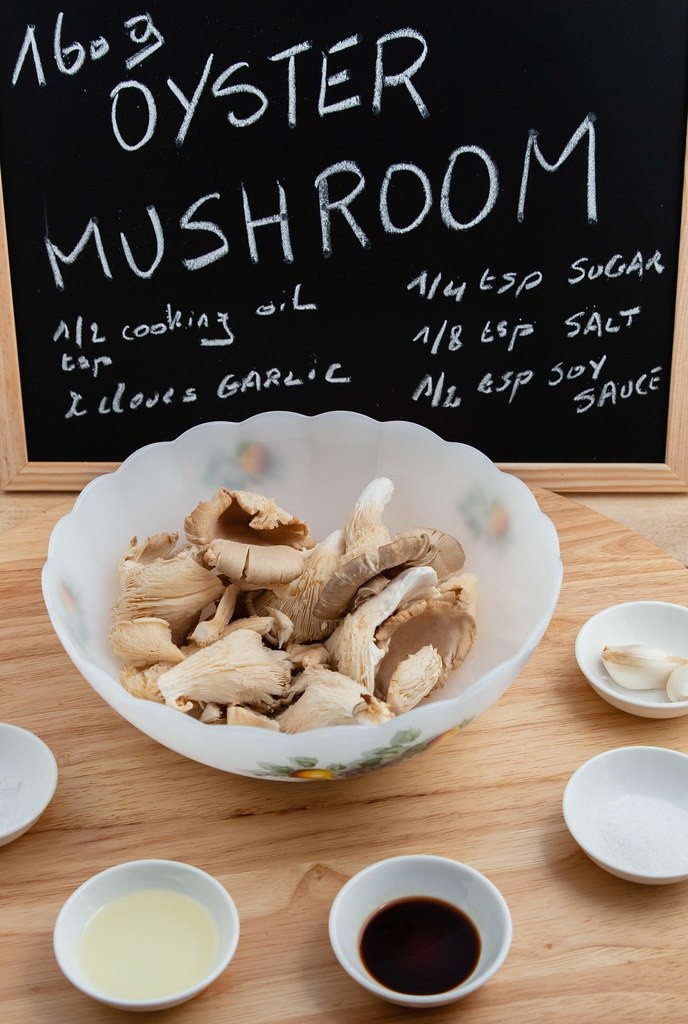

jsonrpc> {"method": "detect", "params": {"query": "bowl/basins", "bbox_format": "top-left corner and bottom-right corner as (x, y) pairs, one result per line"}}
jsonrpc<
(39, 410), (563, 784)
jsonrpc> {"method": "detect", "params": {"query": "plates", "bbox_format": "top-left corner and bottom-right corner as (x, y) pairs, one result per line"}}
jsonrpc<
(53, 859), (241, 1013)
(0, 721), (59, 846)
(562, 745), (688, 886)
(327, 853), (513, 1008)
(574, 600), (688, 719)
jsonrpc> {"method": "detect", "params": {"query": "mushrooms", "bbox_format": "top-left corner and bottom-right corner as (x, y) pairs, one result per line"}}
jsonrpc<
(109, 476), (479, 731)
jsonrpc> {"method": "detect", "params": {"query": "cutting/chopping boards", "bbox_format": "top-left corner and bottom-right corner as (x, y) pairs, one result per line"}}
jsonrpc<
(1, 471), (686, 1022)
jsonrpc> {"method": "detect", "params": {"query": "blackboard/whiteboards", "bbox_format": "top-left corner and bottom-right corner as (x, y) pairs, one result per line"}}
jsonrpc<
(1, 0), (688, 495)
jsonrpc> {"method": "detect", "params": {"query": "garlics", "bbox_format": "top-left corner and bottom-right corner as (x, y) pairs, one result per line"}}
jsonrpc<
(601, 644), (688, 703)
